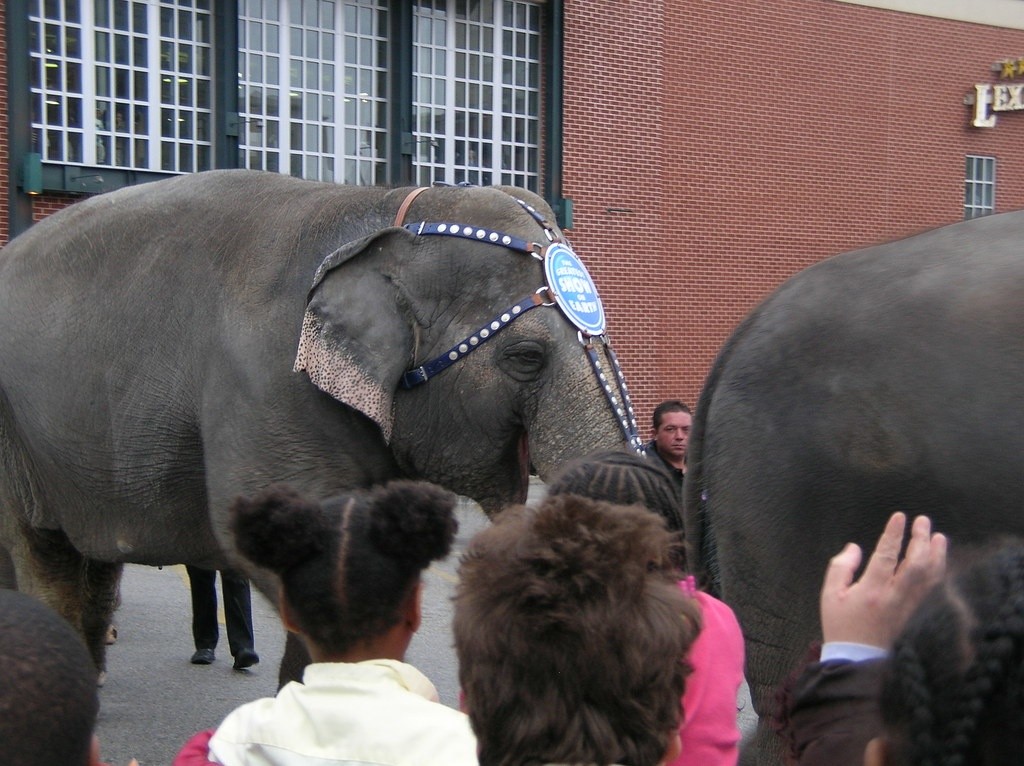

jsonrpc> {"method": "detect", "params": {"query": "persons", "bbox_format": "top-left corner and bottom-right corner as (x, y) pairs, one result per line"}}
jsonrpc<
(170, 481), (478, 766)
(548, 451), (745, 766)
(863, 540), (1024, 766)
(641, 401), (691, 497)
(447, 494), (949, 766)
(186, 565), (259, 669)
(0, 589), (100, 766)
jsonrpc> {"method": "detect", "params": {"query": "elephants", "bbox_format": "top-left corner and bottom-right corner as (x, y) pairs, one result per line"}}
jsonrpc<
(1, 166), (647, 697)
(681, 207), (1023, 766)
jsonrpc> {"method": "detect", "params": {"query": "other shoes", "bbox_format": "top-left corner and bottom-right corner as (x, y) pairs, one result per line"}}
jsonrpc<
(104, 622), (119, 645)
(191, 647), (214, 665)
(232, 649), (258, 669)
